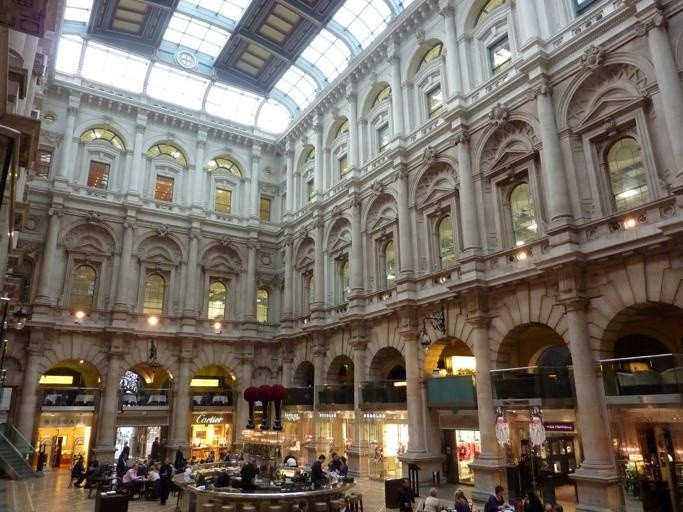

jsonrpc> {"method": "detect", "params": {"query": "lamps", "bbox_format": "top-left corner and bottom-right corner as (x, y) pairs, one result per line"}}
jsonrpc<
(419, 316), (444, 353)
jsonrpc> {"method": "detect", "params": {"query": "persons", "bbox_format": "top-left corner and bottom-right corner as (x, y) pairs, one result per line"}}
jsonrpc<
(206, 449), (244, 487)
(312, 453), (349, 486)
(523, 490), (544, 512)
(338, 496), (351, 512)
(73, 457), (99, 487)
(297, 499), (308, 512)
(398, 479), (442, 512)
(283, 452), (296, 466)
(116, 437), (186, 504)
(455, 488), (470, 512)
(486, 485), (508, 512)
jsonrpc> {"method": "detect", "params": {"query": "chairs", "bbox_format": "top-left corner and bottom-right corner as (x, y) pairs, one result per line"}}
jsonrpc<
(69, 459), (180, 503)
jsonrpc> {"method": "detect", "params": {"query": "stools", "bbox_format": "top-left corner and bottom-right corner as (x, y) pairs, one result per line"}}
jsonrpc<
(201, 493), (363, 512)
